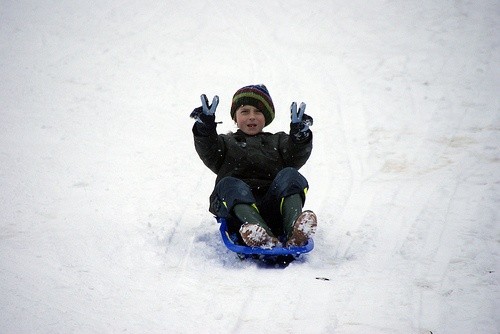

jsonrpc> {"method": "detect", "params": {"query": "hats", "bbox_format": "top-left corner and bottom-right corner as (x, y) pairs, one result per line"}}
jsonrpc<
(231, 85), (276, 127)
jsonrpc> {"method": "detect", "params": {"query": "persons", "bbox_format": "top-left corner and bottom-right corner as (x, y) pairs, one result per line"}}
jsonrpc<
(190, 84), (317, 248)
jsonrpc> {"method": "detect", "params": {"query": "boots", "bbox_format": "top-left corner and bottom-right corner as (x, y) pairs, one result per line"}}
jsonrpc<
(281, 194), (317, 247)
(233, 204), (282, 248)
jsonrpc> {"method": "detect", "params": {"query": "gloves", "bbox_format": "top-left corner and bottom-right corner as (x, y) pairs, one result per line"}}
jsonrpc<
(290, 102), (314, 138)
(190, 93), (219, 126)
